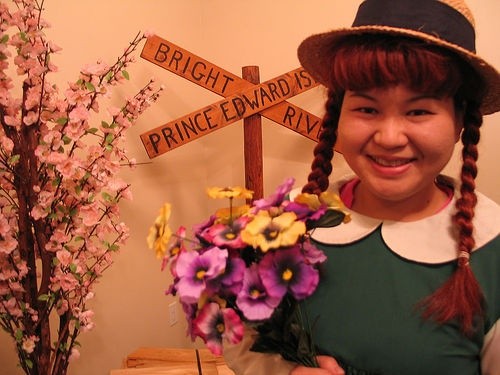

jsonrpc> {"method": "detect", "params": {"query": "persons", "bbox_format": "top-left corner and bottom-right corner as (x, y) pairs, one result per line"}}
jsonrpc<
(223, 0), (500, 375)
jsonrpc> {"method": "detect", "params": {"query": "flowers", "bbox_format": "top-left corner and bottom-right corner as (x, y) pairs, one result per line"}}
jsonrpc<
(0, 0), (165, 375)
(147, 177), (352, 367)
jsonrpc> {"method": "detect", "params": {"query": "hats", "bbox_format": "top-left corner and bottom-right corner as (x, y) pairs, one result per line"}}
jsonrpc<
(297, 0), (500, 116)
